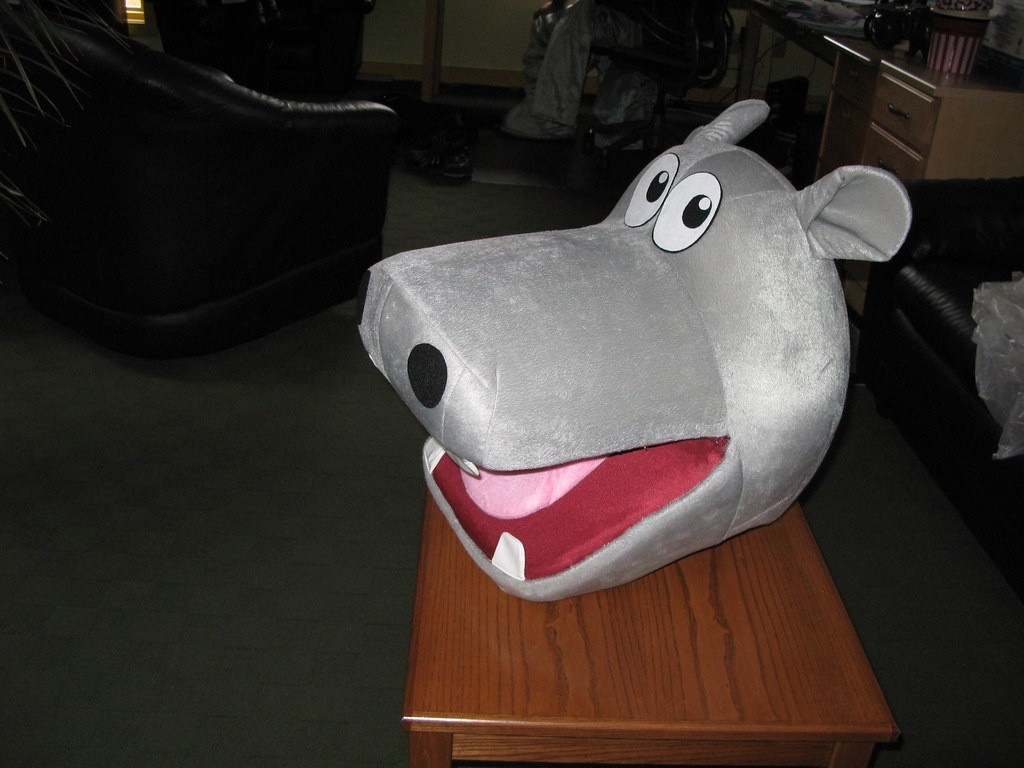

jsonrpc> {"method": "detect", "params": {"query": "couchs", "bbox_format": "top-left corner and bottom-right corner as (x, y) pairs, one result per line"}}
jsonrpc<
(854, 176), (1024, 601)
(0, 0), (400, 357)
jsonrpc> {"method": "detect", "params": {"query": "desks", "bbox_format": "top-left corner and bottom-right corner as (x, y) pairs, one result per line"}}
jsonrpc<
(398, 484), (903, 768)
(737, 0), (1024, 387)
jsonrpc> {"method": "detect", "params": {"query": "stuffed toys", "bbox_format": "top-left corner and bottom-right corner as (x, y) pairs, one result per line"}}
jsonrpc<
(357, 98), (917, 601)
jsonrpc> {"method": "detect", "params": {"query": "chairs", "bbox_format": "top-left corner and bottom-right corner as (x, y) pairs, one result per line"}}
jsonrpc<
(582, 0), (738, 180)
